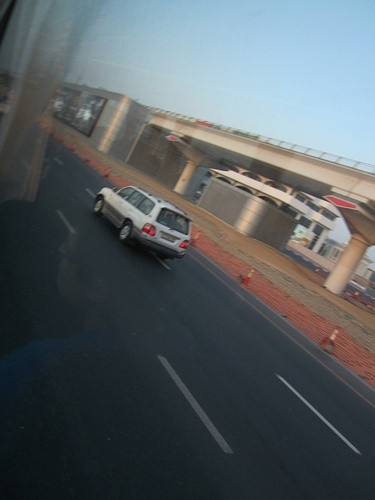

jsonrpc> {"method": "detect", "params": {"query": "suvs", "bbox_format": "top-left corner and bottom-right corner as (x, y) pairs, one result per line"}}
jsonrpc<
(92, 185), (194, 266)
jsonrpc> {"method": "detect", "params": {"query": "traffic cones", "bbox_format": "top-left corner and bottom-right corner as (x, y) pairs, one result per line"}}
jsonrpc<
(190, 230), (201, 249)
(239, 269), (256, 292)
(320, 325), (341, 357)
